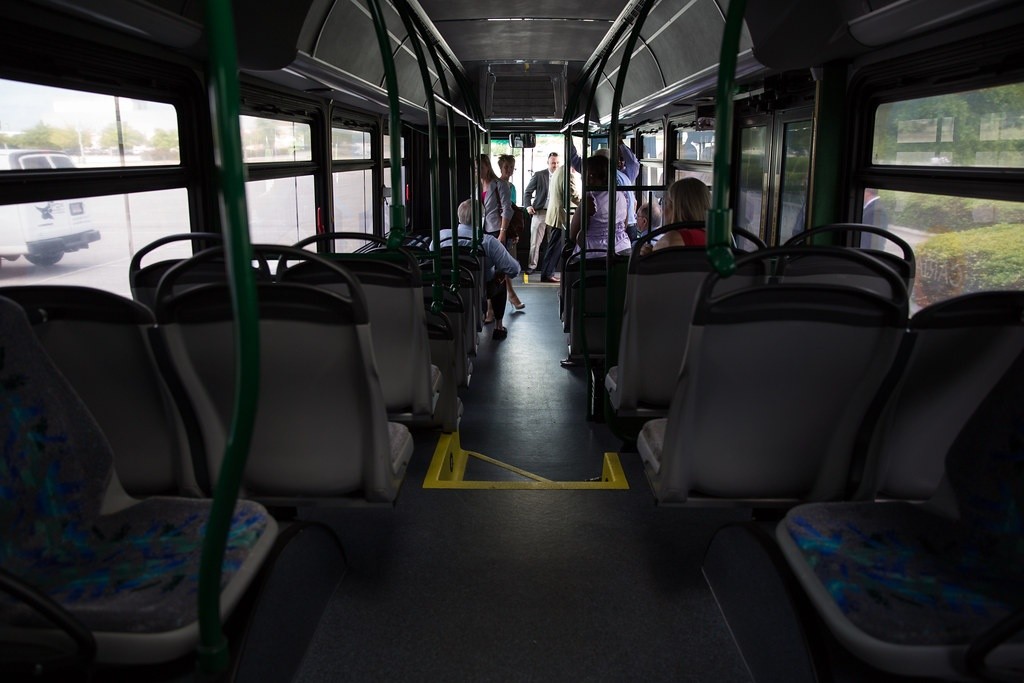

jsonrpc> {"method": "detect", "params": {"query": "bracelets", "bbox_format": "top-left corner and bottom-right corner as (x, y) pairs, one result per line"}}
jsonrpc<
(500, 228), (507, 231)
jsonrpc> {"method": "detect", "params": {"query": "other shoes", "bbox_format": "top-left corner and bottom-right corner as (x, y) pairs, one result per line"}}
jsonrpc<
(525, 268), (535, 275)
(492, 327), (507, 340)
(484, 314), (495, 324)
(560, 357), (579, 367)
(546, 275), (560, 282)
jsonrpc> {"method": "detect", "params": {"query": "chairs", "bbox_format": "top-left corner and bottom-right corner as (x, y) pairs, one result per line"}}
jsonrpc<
(0, 215), (1024, 682)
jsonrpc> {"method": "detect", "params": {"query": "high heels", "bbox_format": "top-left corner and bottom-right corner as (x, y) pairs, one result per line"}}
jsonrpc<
(508, 296), (525, 309)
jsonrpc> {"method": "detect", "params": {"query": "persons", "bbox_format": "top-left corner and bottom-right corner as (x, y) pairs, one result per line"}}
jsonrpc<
(475, 154), (525, 323)
(499, 155), (516, 205)
(541, 164), (582, 281)
(561, 124), (665, 364)
(429, 200), (521, 340)
(524, 152), (560, 273)
(860, 188), (887, 251)
(639, 177), (712, 253)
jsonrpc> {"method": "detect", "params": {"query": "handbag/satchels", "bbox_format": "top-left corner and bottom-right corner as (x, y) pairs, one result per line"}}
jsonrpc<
(496, 179), (524, 239)
